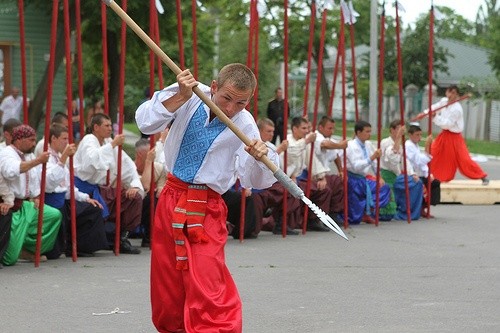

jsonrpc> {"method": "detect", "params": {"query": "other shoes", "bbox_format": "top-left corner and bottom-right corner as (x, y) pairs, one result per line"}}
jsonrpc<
(329, 213), (344, 226)
(120, 239), (141, 254)
(481, 177), (489, 185)
(306, 221), (329, 232)
(420, 204), (433, 218)
(65, 248), (95, 257)
(19, 248), (47, 261)
(273, 225), (300, 236)
(361, 214), (376, 224)
(142, 239), (150, 246)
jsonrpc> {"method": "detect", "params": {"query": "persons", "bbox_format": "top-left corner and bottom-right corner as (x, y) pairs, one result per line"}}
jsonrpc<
(135, 63), (280, 333)
(0, 88), (432, 266)
(417, 84), (490, 185)
